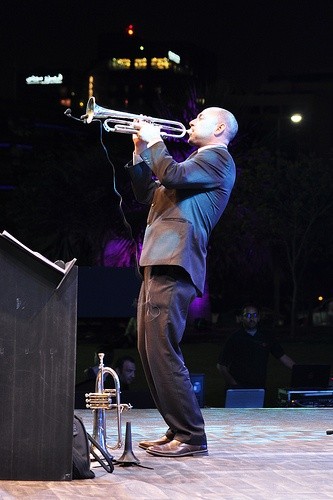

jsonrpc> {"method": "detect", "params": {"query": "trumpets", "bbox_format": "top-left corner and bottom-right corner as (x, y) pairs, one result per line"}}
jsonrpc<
(85, 353), (133, 460)
(86, 97), (193, 139)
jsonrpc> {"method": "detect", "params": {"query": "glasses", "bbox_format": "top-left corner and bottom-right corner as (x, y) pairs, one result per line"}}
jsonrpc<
(244, 313), (257, 318)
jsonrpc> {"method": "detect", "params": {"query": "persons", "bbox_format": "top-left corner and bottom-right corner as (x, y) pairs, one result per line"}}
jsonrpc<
(217, 305), (296, 408)
(122, 107), (238, 457)
(103, 356), (136, 408)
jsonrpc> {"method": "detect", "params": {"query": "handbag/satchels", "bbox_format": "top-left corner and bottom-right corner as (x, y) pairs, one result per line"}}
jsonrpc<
(72, 414), (114, 480)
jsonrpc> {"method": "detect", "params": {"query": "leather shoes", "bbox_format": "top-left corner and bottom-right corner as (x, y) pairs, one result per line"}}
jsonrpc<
(137, 437), (211, 459)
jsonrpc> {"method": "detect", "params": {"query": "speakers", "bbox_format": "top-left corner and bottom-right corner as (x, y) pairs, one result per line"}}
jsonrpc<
(225, 389), (265, 408)
(189, 373), (205, 408)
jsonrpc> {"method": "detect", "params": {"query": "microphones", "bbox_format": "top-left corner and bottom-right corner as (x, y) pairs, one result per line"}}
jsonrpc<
(64, 108), (72, 117)
